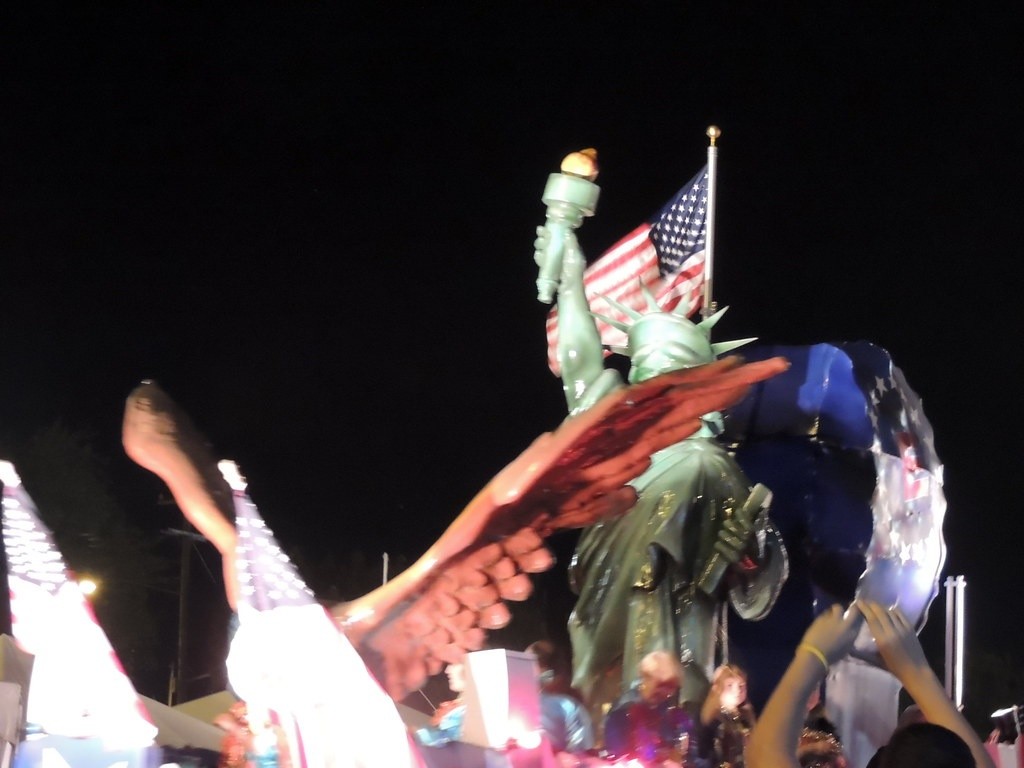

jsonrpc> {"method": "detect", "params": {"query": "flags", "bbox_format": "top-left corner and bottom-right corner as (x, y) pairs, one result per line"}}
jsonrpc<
(2, 481), (158, 751)
(225, 491), (426, 768)
(546, 161), (708, 378)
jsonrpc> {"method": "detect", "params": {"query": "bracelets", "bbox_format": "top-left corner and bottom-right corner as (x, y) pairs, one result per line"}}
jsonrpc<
(795, 644), (829, 677)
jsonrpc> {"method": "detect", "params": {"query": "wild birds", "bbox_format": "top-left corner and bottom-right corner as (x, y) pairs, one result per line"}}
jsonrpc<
(123, 353), (792, 768)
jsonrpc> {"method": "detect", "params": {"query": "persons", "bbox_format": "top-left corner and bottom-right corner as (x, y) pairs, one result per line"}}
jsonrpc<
(606, 653), (692, 768)
(697, 663), (755, 768)
(440, 638), (592, 757)
(534, 223), (788, 750)
(744, 599), (996, 768)
